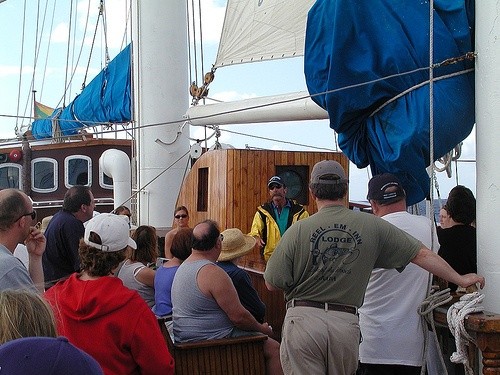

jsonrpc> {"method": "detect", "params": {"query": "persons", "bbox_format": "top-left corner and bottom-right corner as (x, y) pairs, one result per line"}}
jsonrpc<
(0, 188), (104, 375)
(436, 185), (477, 293)
(42, 186), (96, 292)
(41, 213), (175, 375)
(355, 174), (442, 374)
(250, 175), (310, 264)
(171, 219), (284, 375)
(262, 159), (486, 375)
(114, 206), (193, 321)
(215, 228), (266, 325)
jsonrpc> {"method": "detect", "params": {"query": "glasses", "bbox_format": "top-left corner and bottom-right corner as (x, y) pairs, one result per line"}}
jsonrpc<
(14, 211), (36, 223)
(269, 184), (282, 190)
(175, 214), (187, 219)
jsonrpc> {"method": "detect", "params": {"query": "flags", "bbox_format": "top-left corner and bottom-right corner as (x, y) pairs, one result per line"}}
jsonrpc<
(34, 101), (55, 120)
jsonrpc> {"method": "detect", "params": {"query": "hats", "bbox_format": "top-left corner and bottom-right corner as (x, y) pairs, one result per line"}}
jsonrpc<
(268, 176), (285, 186)
(367, 173), (403, 200)
(311, 160), (348, 184)
(216, 228), (256, 261)
(84, 213), (138, 252)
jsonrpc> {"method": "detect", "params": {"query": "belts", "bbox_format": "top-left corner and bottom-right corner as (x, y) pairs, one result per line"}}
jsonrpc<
(163, 317), (172, 322)
(287, 301), (357, 315)
